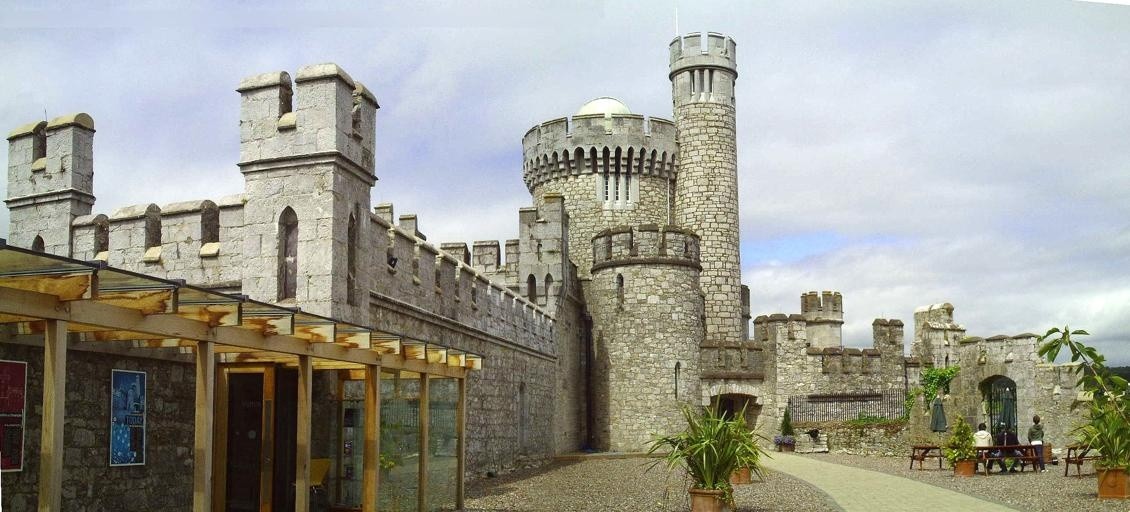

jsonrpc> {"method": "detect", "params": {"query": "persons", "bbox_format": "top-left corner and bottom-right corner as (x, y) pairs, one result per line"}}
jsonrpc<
(974, 423), (994, 473)
(1028, 414), (1046, 473)
(995, 424), (1025, 473)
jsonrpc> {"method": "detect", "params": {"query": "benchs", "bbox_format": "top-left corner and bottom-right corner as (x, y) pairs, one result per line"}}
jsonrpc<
(1060, 455), (1103, 478)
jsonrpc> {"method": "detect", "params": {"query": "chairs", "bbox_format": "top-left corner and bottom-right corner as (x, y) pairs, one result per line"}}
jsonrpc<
(291, 458), (331, 512)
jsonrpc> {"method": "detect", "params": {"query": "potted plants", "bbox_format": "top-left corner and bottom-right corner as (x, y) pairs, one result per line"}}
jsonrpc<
(638, 385), (774, 512)
(943, 414), (978, 476)
(1070, 398), (1130, 499)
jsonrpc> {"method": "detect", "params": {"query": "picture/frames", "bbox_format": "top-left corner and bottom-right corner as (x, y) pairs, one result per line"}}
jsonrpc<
(0, 360), (28, 473)
(109, 370), (147, 467)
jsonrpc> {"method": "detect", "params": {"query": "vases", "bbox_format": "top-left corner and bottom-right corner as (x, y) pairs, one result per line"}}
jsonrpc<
(779, 445), (792, 452)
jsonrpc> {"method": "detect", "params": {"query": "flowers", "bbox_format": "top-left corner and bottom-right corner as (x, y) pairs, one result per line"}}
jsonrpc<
(773, 435), (795, 448)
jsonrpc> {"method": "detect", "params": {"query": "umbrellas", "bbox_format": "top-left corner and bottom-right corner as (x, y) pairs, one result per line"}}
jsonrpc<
(930, 395), (947, 468)
(997, 387), (1016, 430)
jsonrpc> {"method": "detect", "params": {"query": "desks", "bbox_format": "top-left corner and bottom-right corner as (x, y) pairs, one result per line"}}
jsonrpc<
(909, 444), (1040, 476)
(1062, 445), (1103, 478)
